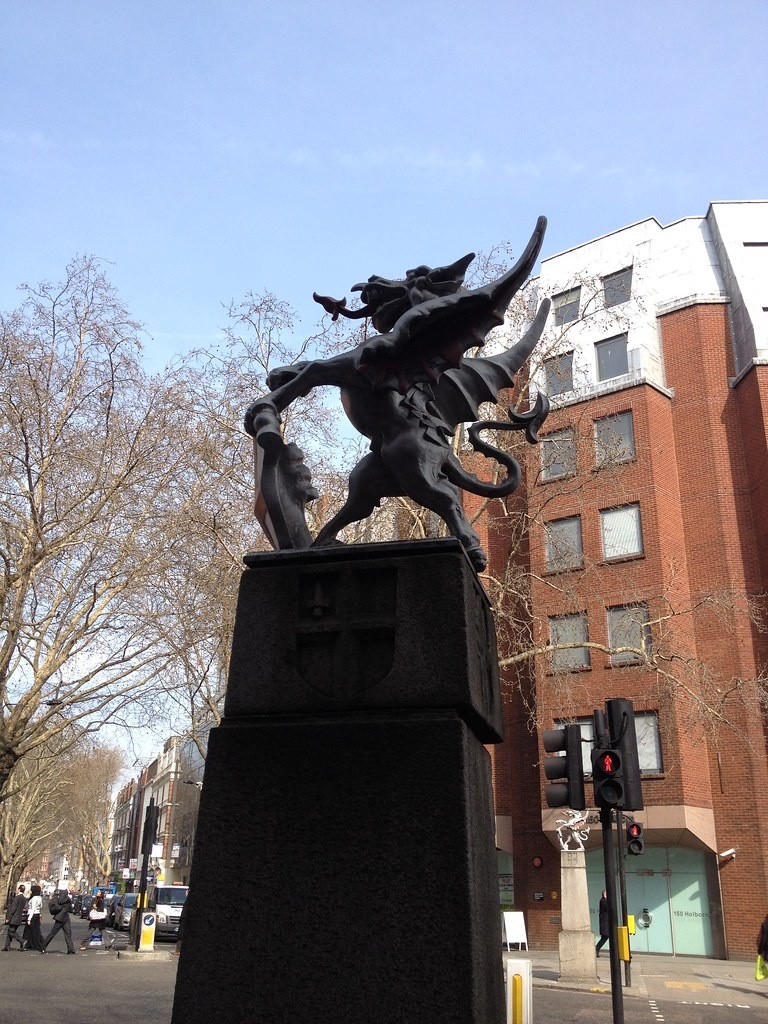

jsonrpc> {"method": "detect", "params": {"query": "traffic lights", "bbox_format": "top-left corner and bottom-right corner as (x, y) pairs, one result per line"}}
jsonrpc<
(540, 724), (586, 812)
(625, 822), (645, 855)
(591, 748), (627, 810)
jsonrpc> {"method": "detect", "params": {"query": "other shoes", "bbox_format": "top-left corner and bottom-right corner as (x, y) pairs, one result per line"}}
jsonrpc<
(105, 945), (112, 951)
(1, 947), (13, 951)
(68, 950), (76, 955)
(41, 949), (47, 953)
(171, 952), (180, 955)
(18, 940), (28, 951)
(79, 946), (88, 951)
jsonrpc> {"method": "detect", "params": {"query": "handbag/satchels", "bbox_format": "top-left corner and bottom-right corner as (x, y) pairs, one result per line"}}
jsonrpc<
(89, 898), (108, 921)
(48, 898), (63, 915)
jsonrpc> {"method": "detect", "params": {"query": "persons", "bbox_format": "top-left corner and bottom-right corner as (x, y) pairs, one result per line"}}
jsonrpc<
(1, 885), (44, 951)
(596, 890), (609, 957)
(756, 915), (768, 962)
(171, 890), (189, 955)
(39, 889), (83, 954)
(80, 890), (112, 951)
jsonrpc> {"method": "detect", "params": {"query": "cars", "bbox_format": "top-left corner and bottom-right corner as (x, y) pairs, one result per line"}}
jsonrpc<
(21, 898), (41, 925)
(70, 882), (191, 942)
